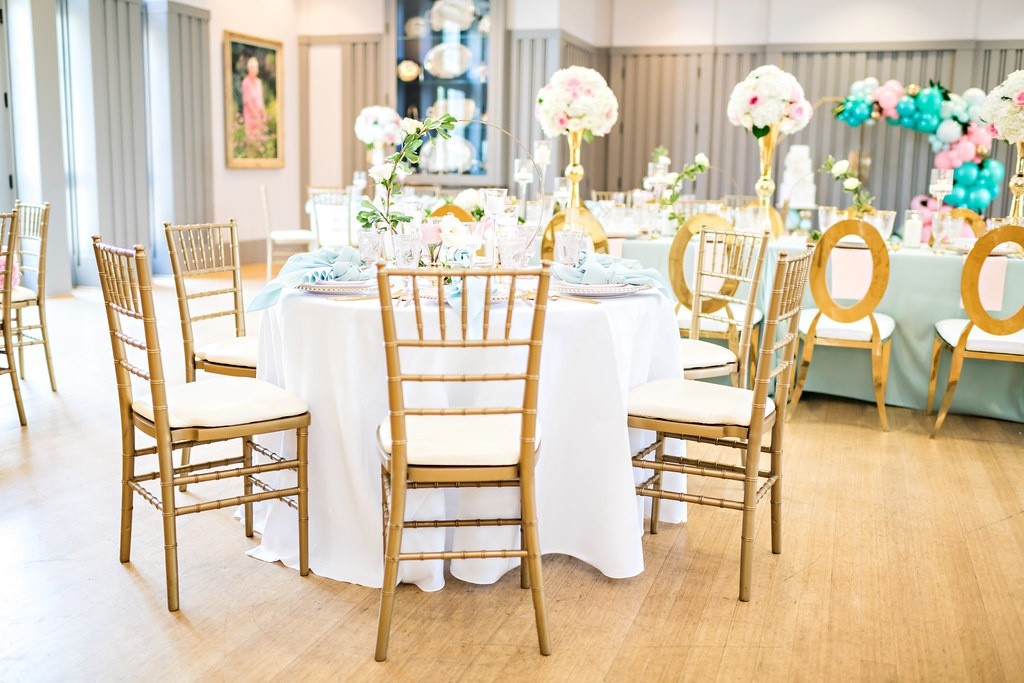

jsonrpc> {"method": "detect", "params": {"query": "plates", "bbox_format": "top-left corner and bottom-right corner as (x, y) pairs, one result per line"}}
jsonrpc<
(952, 236), (1020, 257)
(554, 281), (650, 297)
(413, 285), (533, 305)
(296, 278), (393, 296)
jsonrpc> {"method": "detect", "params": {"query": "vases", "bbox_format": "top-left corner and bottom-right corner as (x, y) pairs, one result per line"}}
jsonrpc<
(1009, 142), (1024, 227)
(742, 124), (784, 240)
(540, 128), (609, 266)
(373, 143), (387, 206)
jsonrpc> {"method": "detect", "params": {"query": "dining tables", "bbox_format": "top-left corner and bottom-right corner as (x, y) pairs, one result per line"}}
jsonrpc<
(234, 277), (689, 593)
(623, 238), (1024, 421)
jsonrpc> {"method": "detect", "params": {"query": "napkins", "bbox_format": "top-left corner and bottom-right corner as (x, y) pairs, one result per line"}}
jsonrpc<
(246, 245), (371, 314)
(550, 250), (679, 320)
(446, 248), (497, 320)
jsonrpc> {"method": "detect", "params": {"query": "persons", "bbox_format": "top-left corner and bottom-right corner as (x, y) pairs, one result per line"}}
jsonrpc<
(241, 57), (268, 152)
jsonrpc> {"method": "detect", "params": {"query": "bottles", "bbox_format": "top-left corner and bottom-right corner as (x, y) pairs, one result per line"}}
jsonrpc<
(454, 185), (583, 281)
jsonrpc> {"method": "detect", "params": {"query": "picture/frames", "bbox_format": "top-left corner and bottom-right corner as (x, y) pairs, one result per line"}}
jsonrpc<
(222, 29), (287, 170)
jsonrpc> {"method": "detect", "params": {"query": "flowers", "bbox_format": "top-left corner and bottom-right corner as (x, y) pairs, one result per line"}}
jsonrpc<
(819, 154), (876, 219)
(0, 246), (21, 291)
(355, 106), (403, 146)
(980, 69), (1024, 145)
(356, 117), (459, 234)
(660, 153), (711, 229)
(536, 66), (619, 137)
(728, 66), (813, 134)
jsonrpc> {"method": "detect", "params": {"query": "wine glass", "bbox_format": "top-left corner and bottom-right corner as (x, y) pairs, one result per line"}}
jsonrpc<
(390, 234), (419, 298)
(353, 171), (367, 196)
(592, 189), (674, 233)
(928, 170), (965, 249)
(513, 141), (573, 232)
(818, 205), (896, 243)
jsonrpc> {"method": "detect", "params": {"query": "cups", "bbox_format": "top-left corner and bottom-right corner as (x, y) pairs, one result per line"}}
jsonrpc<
(356, 229), (384, 265)
(345, 185), (415, 210)
(903, 210), (924, 247)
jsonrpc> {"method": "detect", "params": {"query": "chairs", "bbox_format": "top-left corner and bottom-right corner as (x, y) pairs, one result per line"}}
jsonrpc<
(0, 210), (28, 426)
(92, 235), (312, 612)
(422, 203), (485, 257)
(308, 187), (352, 248)
(260, 185), (311, 281)
(675, 196), (758, 212)
(541, 207), (609, 267)
(627, 244), (817, 603)
(0, 199), (57, 391)
(590, 189), (636, 208)
(375, 261), (554, 663)
(927, 226), (1024, 438)
(668, 213), (763, 391)
(680, 229), (770, 467)
(786, 218), (897, 432)
(163, 219), (256, 492)
